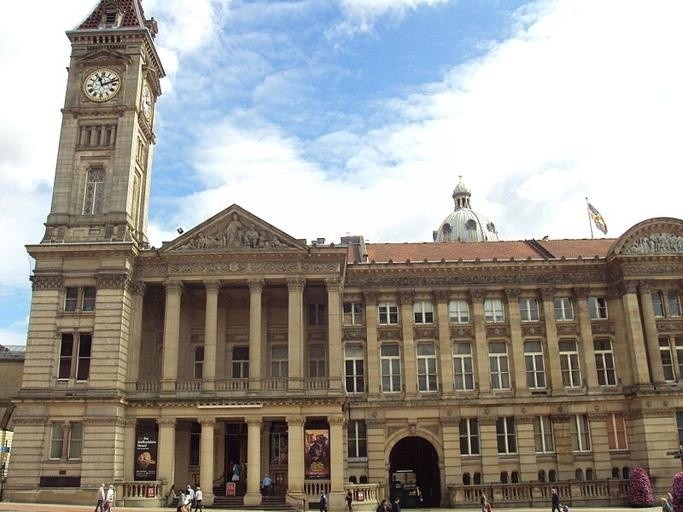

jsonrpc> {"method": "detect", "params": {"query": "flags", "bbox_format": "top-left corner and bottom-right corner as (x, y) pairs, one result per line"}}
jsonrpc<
(588, 204), (608, 235)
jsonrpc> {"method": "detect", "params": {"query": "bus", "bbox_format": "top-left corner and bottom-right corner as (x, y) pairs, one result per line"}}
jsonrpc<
(389, 464), (417, 510)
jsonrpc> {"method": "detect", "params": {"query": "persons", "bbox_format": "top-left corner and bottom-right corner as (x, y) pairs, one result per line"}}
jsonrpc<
(661, 498), (673, 512)
(376, 497), (401, 512)
(561, 503), (568, 512)
(345, 488), (353, 512)
(479, 493), (486, 512)
(551, 488), (561, 512)
(667, 492), (673, 506)
(95, 483), (115, 512)
(320, 489), (328, 512)
(227, 461), (247, 482)
(262, 473), (272, 500)
(414, 485), (422, 505)
(167, 484), (203, 512)
(172, 214), (288, 249)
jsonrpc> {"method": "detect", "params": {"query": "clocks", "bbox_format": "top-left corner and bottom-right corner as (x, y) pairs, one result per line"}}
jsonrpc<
(138, 81), (155, 123)
(80, 65), (121, 103)
